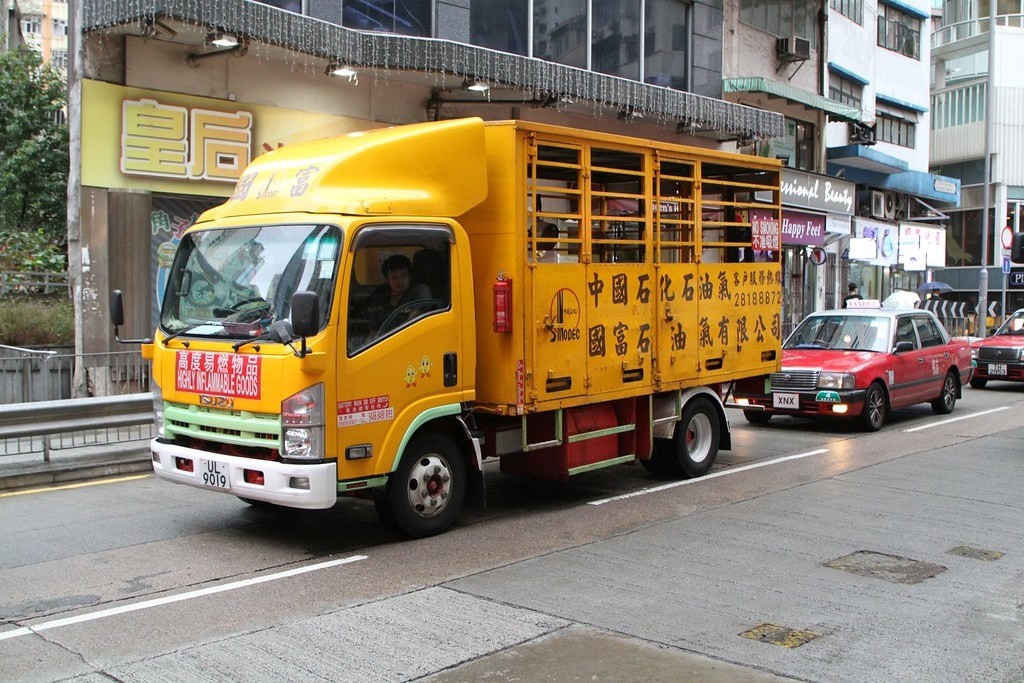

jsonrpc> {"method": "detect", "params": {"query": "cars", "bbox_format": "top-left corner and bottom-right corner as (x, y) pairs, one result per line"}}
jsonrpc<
(969, 308), (1024, 389)
(736, 300), (972, 431)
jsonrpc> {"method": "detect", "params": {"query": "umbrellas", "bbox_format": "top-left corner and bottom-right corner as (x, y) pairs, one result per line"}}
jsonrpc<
(914, 281), (953, 301)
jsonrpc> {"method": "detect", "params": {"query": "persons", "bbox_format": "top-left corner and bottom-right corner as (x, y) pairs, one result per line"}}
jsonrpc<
(530, 220), (559, 252)
(841, 283), (864, 309)
(928, 289), (943, 300)
(364, 255), (432, 348)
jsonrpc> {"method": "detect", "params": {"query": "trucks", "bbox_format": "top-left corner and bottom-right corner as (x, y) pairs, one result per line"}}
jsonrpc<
(109, 116), (783, 539)
